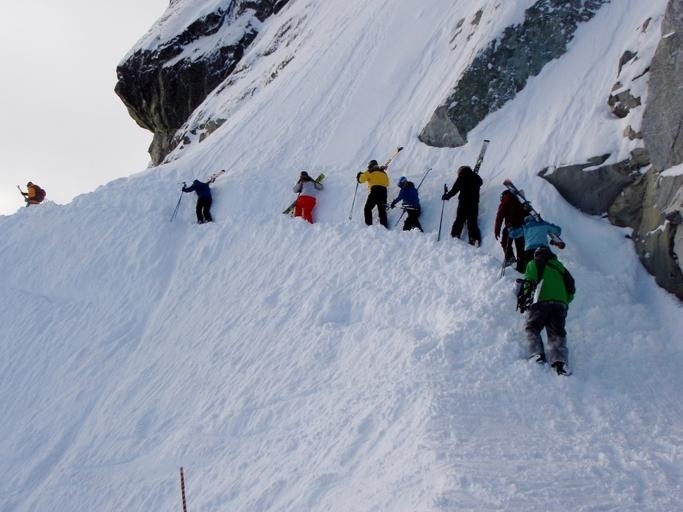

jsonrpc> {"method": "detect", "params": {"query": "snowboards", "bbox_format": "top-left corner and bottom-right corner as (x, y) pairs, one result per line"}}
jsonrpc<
(281, 174), (325, 214)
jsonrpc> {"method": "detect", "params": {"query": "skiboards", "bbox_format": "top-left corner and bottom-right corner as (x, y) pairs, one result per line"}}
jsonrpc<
(380, 147), (403, 170)
(472, 140), (490, 175)
(207, 169), (225, 183)
(503, 179), (565, 249)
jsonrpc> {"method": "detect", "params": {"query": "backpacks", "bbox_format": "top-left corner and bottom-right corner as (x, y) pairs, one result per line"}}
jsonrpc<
(30, 185), (45, 202)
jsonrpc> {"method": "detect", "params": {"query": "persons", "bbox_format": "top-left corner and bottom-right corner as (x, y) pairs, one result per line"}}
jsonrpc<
(390, 176), (424, 232)
(355, 159), (390, 230)
(290, 170), (322, 225)
(180, 179), (215, 225)
(492, 188), (527, 274)
(441, 165), (483, 249)
(19, 180), (46, 208)
(503, 214), (562, 281)
(509, 244), (576, 376)
